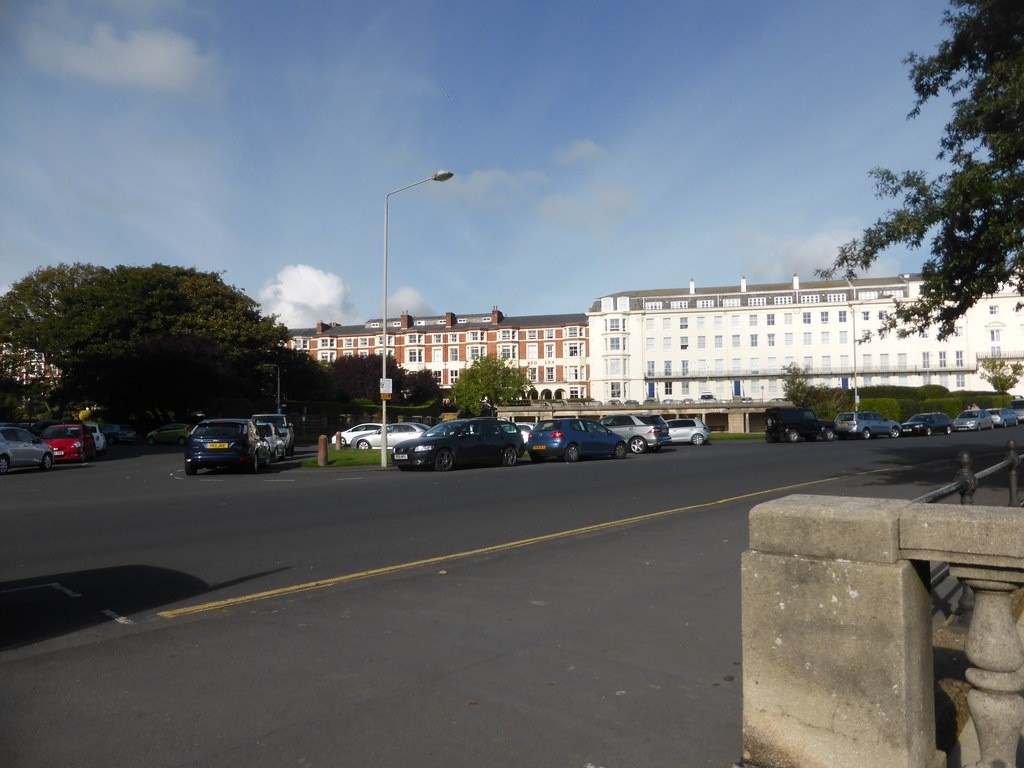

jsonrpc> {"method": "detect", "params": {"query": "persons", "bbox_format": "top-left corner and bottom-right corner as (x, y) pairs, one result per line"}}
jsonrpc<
(967, 402), (979, 410)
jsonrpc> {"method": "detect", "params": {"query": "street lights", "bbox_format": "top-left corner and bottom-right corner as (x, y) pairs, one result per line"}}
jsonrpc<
(263, 364), (282, 413)
(852, 295), (894, 411)
(380, 171), (455, 467)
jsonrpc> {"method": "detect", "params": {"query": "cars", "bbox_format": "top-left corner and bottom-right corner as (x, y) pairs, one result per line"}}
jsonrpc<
(666, 418), (709, 445)
(0, 426), (54, 474)
(509, 391), (786, 406)
(145, 423), (194, 445)
(350, 422), (455, 450)
(68, 425), (107, 454)
(526, 419), (628, 462)
(257, 423), (288, 463)
(902, 413), (955, 436)
(952, 409), (994, 432)
(42, 424), (96, 463)
(331, 422), (388, 447)
(986, 408), (1019, 429)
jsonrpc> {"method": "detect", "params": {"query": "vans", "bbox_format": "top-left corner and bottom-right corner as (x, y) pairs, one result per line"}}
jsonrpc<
(1010, 396), (1024, 422)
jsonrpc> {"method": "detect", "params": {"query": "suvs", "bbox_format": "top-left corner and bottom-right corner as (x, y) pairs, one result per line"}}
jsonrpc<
(598, 414), (671, 454)
(184, 419), (272, 475)
(251, 413), (296, 456)
(764, 406), (836, 443)
(391, 417), (526, 471)
(834, 411), (902, 439)
(502, 422), (539, 445)
(99, 423), (137, 445)
